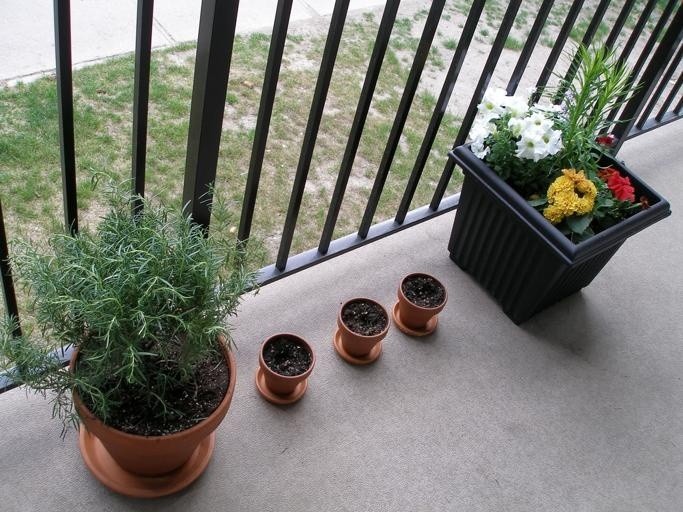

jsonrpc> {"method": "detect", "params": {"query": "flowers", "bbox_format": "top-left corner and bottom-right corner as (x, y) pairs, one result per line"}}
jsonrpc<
(472, 38), (652, 242)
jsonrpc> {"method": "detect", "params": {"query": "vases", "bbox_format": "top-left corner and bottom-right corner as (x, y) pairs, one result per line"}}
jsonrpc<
(256, 275), (448, 404)
(449, 143), (672, 325)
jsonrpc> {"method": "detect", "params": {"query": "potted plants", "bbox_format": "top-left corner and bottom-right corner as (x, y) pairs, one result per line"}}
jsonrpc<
(0, 178), (269, 500)
